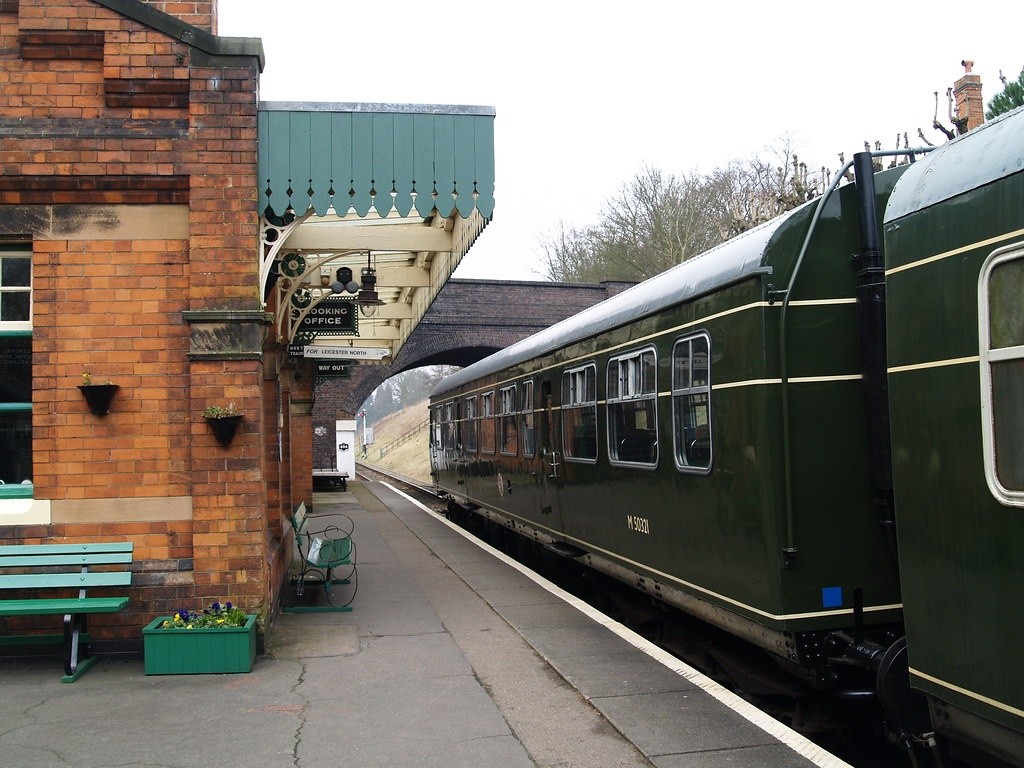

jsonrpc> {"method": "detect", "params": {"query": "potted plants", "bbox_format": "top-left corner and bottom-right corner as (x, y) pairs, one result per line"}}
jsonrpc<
(202, 405), (244, 447)
(76, 372), (120, 417)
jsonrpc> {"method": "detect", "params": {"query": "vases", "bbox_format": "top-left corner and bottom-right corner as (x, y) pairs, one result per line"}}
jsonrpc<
(142, 614), (258, 676)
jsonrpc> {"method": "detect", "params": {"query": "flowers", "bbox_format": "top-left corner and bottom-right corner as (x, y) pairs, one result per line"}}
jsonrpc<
(159, 600), (249, 629)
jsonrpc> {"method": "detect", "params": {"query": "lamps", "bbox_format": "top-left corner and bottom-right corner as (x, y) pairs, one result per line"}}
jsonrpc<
(351, 250), (387, 318)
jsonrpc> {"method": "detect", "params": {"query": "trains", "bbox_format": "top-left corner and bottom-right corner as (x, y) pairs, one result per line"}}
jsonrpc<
(428, 106), (1024, 767)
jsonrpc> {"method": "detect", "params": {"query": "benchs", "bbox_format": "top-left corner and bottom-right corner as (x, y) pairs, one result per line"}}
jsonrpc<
(0, 541), (134, 683)
(282, 500), (359, 613)
